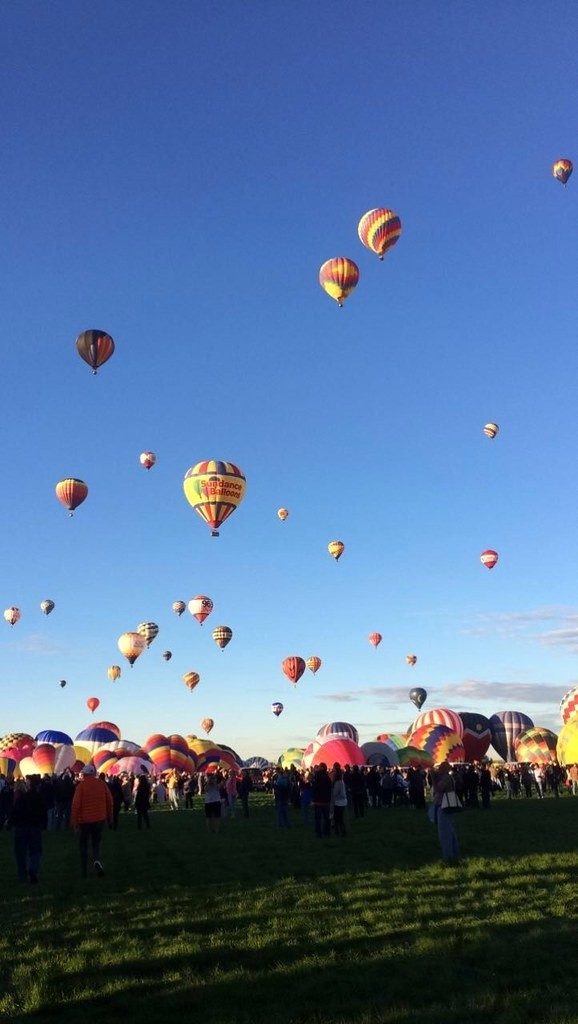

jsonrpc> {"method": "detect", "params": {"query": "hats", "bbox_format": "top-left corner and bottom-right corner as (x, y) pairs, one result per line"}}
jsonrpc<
(81, 765), (95, 774)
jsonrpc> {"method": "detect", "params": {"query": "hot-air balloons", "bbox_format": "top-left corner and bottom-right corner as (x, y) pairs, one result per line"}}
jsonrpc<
(136, 621), (160, 650)
(40, 599), (55, 618)
(367, 632), (383, 648)
(483, 422), (500, 441)
(210, 626), (234, 652)
(4, 606), (21, 630)
(278, 508), (288, 523)
(172, 600), (187, 621)
(76, 329), (116, 375)
(54, 477), (88, 517)
(139, 450), (157, 472)
(189, 595), (214, 628)
(183, 460), (246, 537)
(0, 650), (578, 782)
(319, 258), (359, 307)
(358, 208), (402, 261)
(552, 158), (575, 189)
(117, 632), (147, 668)
(327, 540), (344, 563)
(481, 550), (499, 570)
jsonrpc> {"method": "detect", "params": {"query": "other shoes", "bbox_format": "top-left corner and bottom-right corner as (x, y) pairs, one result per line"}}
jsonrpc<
(94, 860), (104, 877)
(20, 868), (39, 883)
(81, 866), (87, 878)
(335, 830), (346, 836)
(316, 832), (331, 838)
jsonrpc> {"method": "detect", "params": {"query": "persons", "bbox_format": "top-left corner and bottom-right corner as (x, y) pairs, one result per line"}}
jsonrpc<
(6, 774), (48, 884)
(70, 765), (114, 876)
(432, 762), (466, 864)
(0, 760), (578, 869)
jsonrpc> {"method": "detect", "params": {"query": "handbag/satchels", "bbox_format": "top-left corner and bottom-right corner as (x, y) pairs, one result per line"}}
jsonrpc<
(441, 791), (462, 809)
